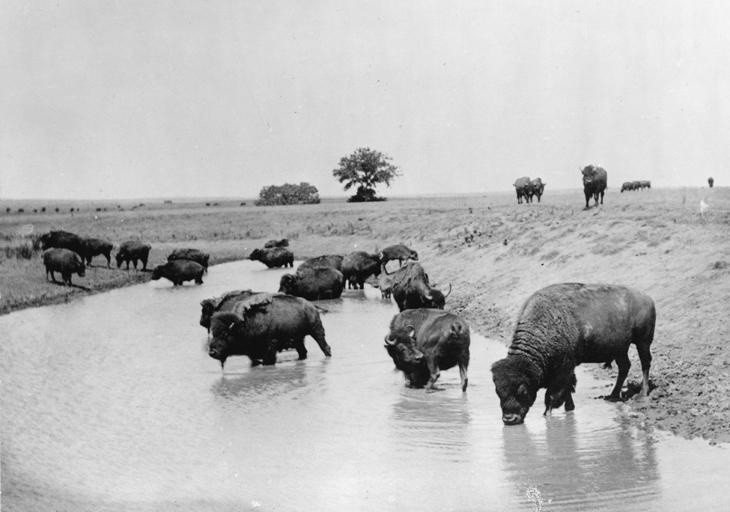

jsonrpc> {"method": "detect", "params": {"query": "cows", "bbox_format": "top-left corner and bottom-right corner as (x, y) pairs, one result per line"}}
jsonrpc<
(115, 241), (151, 271)
(384, 307), (470, 392)
(247, 246), (295, 269)
(620, 179), (651, 193)
(40, 246), (86, 285)
(277, 242), (454, 314)
(167, 248), (209, 274)
(490, 282), (656, 427)
(150, 259), (205, 287)
(513, 177), (545, 204)
(264, 238), (293, 249)
(579, 165), (608, 209)
(36, 230), (113, 269)
(199, 289), (332, 368)
